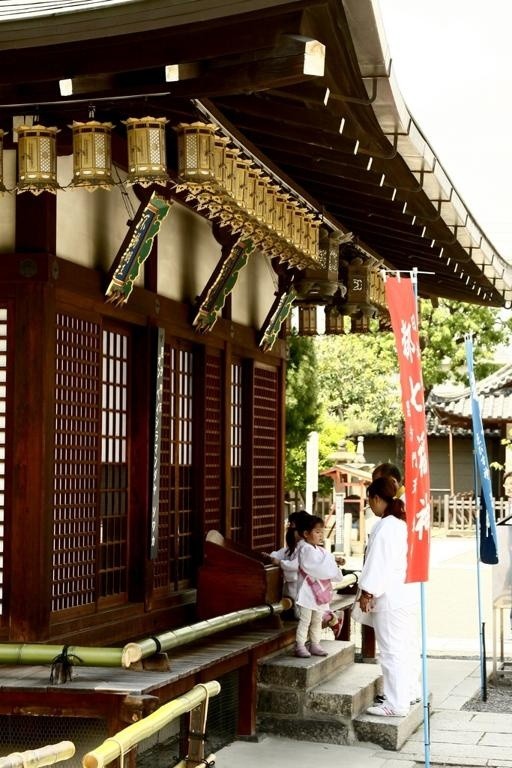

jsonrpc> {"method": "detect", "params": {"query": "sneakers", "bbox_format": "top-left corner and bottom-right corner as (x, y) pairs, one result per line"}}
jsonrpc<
(295, 644), (328, 658)
(328, 613), (343, 638)
(366, 695), (422, 716)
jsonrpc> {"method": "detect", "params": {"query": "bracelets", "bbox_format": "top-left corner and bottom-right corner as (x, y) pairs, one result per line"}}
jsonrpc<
(362, 590), (373, 599)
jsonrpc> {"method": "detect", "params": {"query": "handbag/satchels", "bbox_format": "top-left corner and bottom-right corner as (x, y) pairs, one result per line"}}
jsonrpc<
(311, 579), (334, 605)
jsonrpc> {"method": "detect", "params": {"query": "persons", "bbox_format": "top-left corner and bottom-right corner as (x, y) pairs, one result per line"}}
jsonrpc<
(358, 477), (420, 718)
(285, 515), (343, 658)
(268, 511), (337, 641)
(350, 464), (422, 705)
